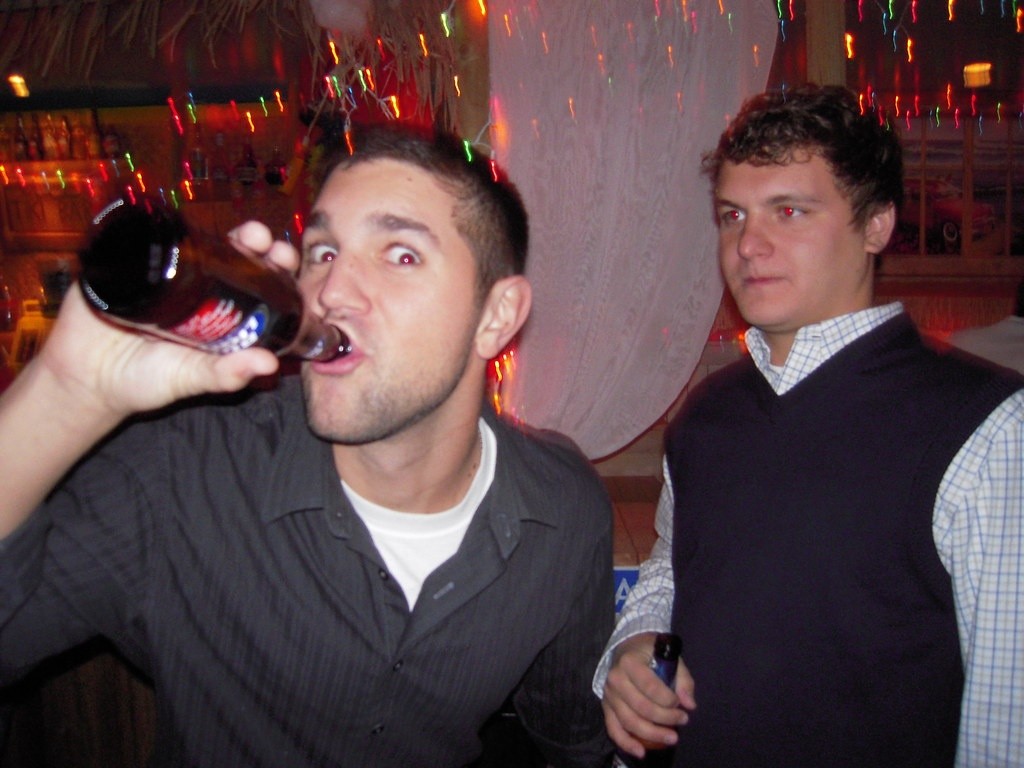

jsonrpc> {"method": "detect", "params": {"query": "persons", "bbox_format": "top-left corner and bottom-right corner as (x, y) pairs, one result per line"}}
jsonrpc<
(592, 83), (1024, 768)
(1, 122), (618, 768)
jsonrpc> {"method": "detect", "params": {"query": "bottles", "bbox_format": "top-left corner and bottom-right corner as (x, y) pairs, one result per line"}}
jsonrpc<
(187, 123), (282, 188)
(13, 108), (102, 163)
(607, 632), (685, 768)
(77, 198), (351, 366)
